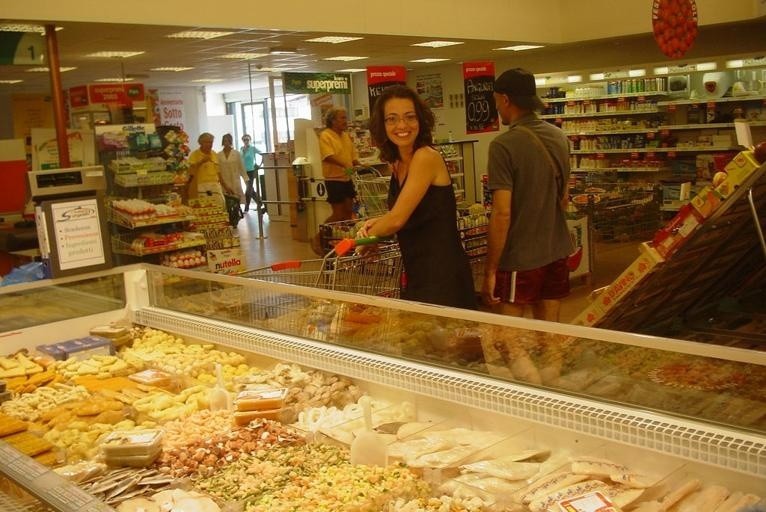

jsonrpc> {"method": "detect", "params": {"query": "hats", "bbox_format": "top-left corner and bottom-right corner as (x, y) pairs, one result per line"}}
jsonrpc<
(493, 68), (547, 110)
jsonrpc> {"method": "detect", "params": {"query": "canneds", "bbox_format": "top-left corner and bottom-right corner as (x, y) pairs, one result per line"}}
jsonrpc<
(223, 237), (239, 248)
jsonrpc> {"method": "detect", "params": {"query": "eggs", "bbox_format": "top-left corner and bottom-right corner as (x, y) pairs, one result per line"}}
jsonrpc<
(111, 198), (175, 222)
(160, 249), (206, 269)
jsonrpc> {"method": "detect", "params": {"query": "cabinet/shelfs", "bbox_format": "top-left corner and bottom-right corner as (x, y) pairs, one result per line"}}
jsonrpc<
(0, 262), (764, 512)
(93, 122), (207, 273)
(537, 95), (766, 172)
(432, 136), (478, 207)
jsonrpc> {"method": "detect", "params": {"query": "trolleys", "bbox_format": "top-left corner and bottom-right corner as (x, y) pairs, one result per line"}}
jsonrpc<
(317, 161), (506, 300)
(208, 235), (406, 341)
(578, 169), (663, 246)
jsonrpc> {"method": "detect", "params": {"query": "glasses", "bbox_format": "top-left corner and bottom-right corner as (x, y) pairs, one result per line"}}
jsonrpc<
(386, 113), (417, 126)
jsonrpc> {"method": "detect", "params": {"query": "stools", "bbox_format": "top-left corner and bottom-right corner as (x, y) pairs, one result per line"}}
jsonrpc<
(8, 248), (40, 263)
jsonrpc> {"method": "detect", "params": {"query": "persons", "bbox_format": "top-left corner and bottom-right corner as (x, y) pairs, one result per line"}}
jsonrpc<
(217, 134), (249, 228)
(188, 133), (233, 200)
(354, 84), (488, 372)
(239, 134), (261, 210)
(311, 105), (360, 256)
(480, 67), (571, 384)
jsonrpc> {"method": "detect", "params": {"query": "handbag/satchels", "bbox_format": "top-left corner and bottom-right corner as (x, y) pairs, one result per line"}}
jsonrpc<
(224, 192), (243, 227)
